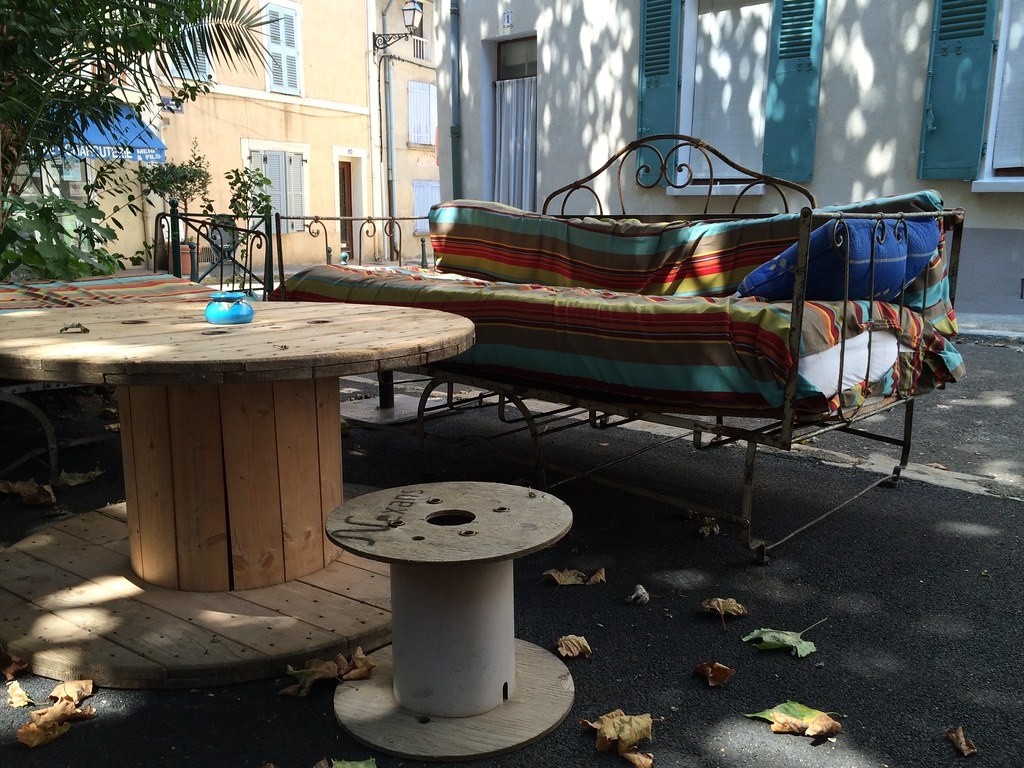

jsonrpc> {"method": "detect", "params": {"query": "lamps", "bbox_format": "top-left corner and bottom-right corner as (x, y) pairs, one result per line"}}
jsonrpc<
(373, 0), (423, 56)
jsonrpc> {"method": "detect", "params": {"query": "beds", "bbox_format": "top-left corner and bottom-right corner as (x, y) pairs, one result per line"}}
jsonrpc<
(0, 198), (274, 314)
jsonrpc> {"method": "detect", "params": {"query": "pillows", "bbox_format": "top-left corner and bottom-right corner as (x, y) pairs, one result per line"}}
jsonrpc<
(737, 219), (941, 302)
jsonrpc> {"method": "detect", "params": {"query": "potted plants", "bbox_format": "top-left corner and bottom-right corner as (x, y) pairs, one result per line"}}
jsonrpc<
(139, 137), (212, 274)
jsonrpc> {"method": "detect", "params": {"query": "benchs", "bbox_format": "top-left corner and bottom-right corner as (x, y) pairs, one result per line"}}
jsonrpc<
(268, 134), (971, 564)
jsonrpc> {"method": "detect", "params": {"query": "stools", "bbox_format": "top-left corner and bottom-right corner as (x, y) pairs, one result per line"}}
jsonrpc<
(324, 481), (575, 764)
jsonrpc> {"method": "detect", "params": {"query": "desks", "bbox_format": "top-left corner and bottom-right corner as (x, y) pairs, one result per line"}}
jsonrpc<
(1, 297), (477, 694)
